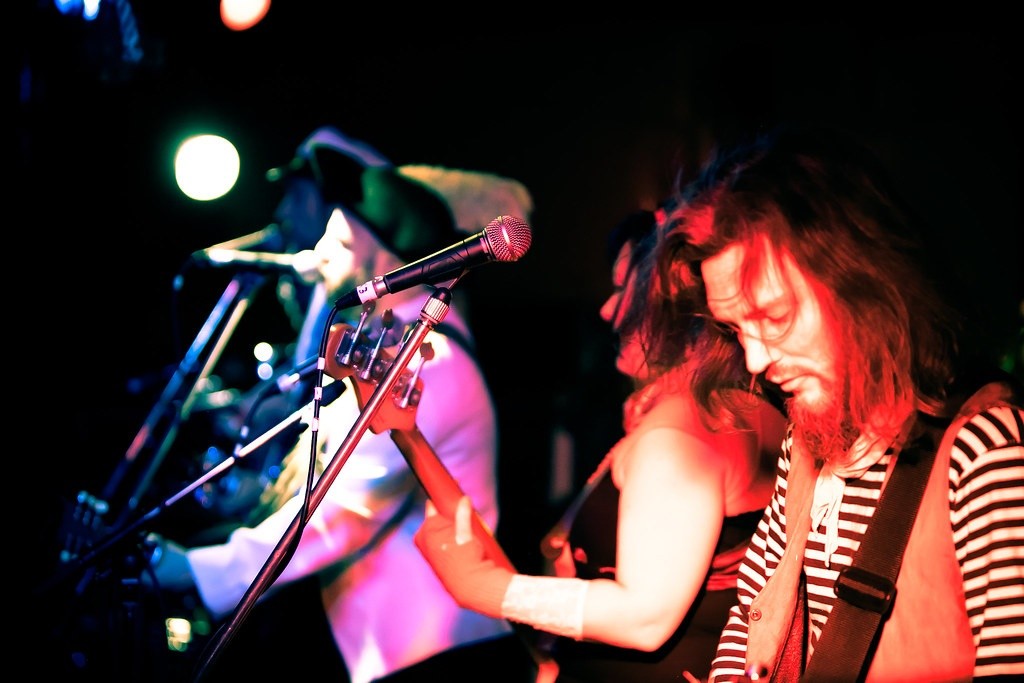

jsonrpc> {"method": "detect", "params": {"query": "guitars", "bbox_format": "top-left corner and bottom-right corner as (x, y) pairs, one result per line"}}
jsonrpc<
(324, 300), (577, 683)
(61, 489), (165, 579)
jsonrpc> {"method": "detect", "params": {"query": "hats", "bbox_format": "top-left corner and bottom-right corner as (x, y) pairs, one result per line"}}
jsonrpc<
(302, 123), (533, 281)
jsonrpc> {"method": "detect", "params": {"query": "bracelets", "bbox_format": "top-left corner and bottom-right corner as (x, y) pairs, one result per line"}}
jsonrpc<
(502, 574), (587, 638)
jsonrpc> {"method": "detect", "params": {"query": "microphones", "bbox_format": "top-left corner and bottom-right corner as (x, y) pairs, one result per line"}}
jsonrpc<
(191, 223), (283, 262)
(335, 215), (531, 313)
(206, 248), (320, 282)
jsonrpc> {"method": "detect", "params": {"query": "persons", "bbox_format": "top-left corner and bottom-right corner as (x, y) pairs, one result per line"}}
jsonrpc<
(114, 165), (531, 683)
(416, 145), (1024, 683)
